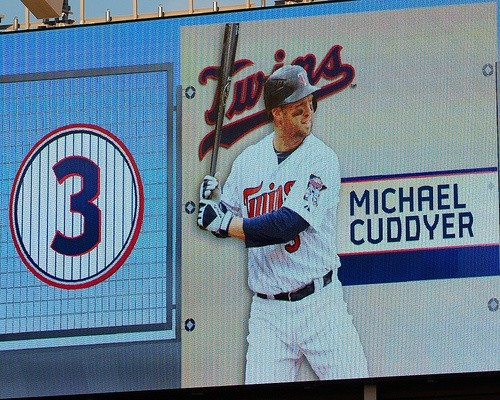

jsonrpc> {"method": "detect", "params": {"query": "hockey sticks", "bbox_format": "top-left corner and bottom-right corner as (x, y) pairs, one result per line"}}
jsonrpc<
(197, 21), (241, 231)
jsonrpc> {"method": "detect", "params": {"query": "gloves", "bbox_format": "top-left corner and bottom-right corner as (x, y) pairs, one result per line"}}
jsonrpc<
(199, 171), (228, 215)
(197, 199), (233, 238)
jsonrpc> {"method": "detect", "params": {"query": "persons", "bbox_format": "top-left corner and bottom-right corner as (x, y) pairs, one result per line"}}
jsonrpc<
(197, 64), (370, 385)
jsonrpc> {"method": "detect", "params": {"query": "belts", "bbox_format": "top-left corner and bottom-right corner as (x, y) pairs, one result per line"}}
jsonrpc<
(257, 270), (333, 302)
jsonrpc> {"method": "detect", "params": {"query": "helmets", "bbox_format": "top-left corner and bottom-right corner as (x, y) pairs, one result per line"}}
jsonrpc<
(264, 65), (321, 111)
